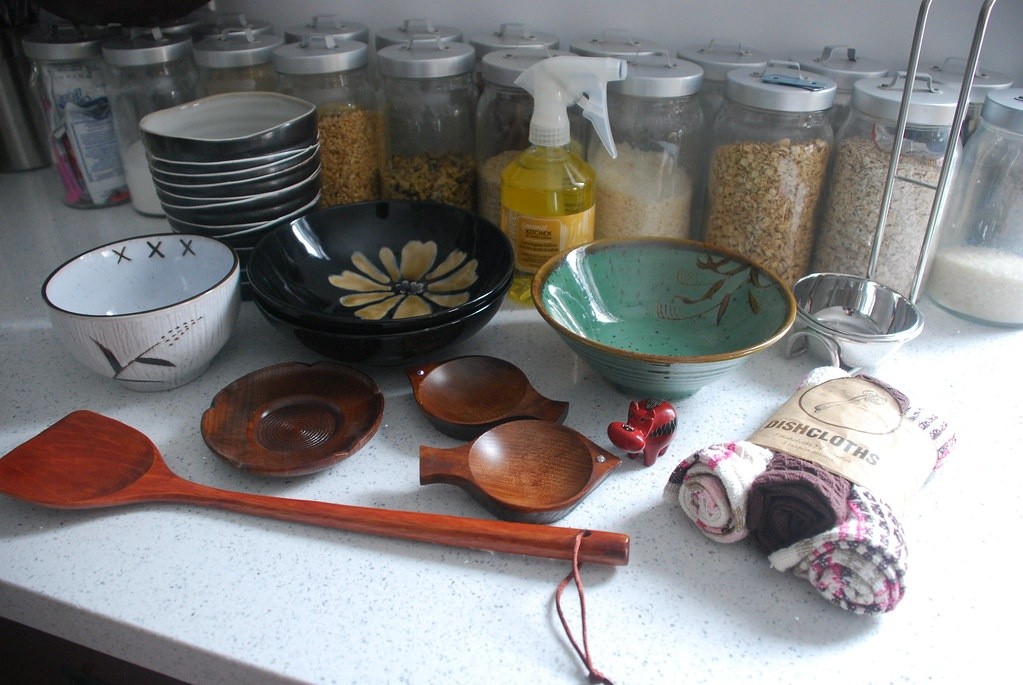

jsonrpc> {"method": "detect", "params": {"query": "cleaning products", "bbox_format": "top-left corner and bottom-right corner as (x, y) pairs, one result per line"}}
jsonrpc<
(499, 52), (629, 311)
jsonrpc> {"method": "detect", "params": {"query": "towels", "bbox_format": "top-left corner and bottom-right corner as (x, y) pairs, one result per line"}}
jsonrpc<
(663, 363), (961, 619)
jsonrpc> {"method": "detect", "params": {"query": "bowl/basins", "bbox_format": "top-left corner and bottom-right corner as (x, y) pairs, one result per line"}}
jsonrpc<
(41, 232), (241, 393)
(793, 272), (926, 370)
(530, 235), (797, 401)
(139, 91), (322, 302)
(246, 198), (515, 361)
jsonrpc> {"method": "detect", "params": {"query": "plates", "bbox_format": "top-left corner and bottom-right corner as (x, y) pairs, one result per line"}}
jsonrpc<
(200, 361), (384, 477)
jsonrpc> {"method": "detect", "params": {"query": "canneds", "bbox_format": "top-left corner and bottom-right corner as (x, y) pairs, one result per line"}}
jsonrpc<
(14, 13), (1023, 329)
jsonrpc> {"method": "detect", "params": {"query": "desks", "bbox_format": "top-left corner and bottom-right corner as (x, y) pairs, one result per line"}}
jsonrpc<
(0, 170), (1023, 685)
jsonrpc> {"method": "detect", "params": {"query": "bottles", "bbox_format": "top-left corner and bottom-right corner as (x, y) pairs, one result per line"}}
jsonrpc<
(925, 87), (1023, 324)
(0, 34), (48, 172)
(374, 33), (479, 214)
(20, 30), (131, 210)
(192, 17), (286, 103)
(807, 71), (969, 303)
(100, 16), (197, 217)
(284, 15), (370, 45)
(677, 38), (772, 120)
(787, 44), (890, 138)
(700, 59), (838, 293)
(376, 18), (464, 92)
(469, 22), (582, 226)
(920, 56), (1014, 148)
(569, 28), (711, 242)
(273, 40), (377, 209)
